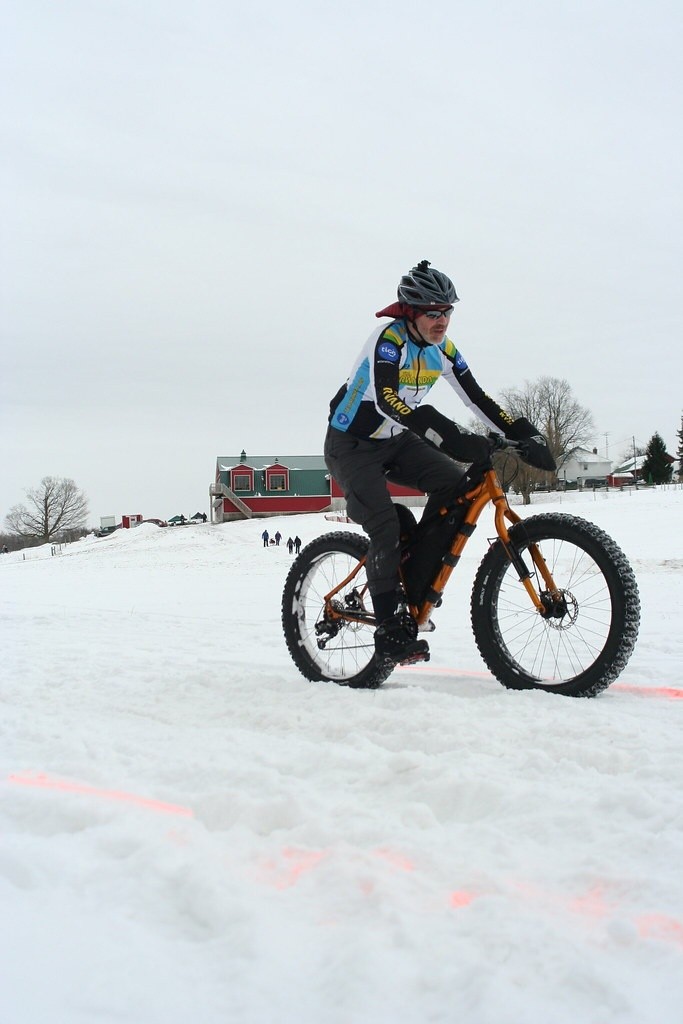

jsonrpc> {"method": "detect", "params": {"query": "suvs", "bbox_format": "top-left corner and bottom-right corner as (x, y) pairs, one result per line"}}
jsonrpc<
(97, 525), (117, 538)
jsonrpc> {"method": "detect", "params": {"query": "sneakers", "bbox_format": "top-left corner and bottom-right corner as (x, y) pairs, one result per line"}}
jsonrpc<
(374, 615), (429, 671)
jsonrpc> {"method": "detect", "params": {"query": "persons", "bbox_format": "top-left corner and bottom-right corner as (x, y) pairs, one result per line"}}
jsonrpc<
(275, 531), (281, 546)
(287, 537), (294, 553)
(325, 261), (517, 666)
(262, 530), (269, 547)
(294, 536), (301, 554)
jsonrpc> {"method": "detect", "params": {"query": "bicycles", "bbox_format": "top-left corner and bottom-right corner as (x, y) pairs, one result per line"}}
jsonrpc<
(280, 429), (642, 701)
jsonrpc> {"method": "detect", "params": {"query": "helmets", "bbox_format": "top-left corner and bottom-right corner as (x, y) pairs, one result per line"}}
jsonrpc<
(397, 266), (460, 305)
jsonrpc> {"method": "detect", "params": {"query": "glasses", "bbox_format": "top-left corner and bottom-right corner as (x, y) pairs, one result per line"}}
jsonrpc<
(411, 306), (454, 320)
